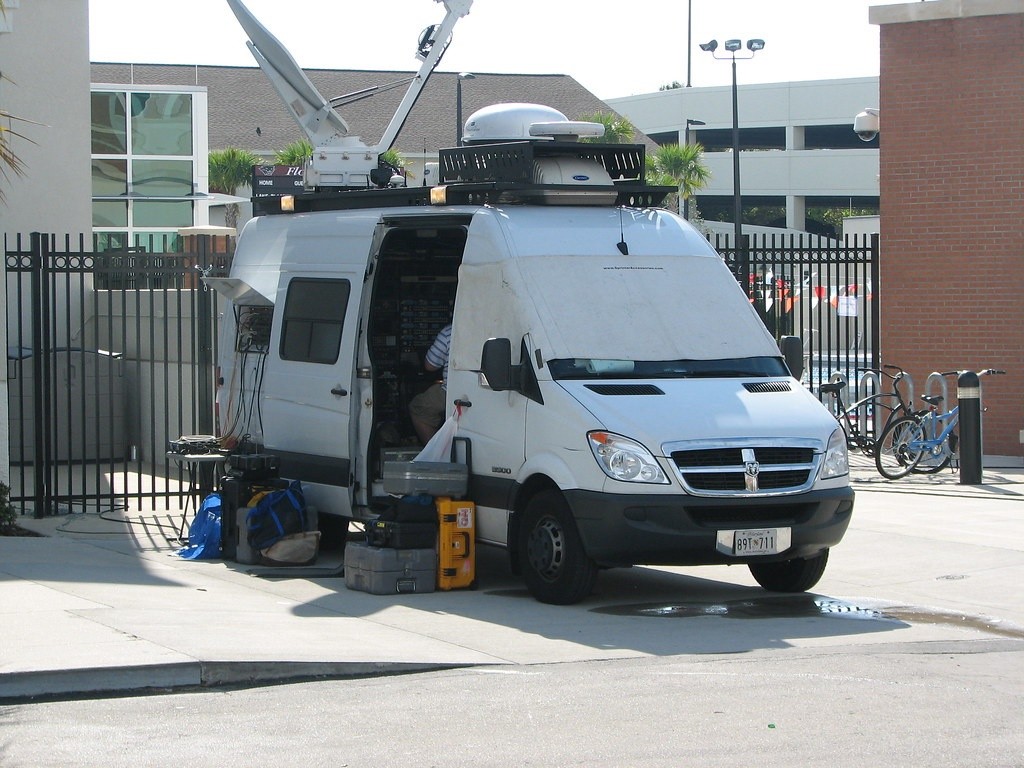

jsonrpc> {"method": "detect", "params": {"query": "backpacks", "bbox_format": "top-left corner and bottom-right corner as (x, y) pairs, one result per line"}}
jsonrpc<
(177, 494), (222, 560)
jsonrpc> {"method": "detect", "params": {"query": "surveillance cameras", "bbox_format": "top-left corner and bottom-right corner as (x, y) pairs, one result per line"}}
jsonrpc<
(855, 112), (879, 142)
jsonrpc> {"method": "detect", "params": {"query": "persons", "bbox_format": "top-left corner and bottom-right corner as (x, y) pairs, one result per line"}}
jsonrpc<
(407, 324), (452, 446)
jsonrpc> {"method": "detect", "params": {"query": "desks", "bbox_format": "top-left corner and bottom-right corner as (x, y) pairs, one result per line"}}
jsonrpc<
(166, 451), (225, 547)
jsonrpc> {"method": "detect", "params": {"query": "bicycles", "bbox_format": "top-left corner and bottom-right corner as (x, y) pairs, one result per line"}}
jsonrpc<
(816, 365), (1009, 480)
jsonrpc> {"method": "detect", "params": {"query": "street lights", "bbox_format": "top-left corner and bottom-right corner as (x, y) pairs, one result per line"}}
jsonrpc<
(455, 72), (474, 161)
(701, 40), (765, 287)
(684, 120), (706, 222)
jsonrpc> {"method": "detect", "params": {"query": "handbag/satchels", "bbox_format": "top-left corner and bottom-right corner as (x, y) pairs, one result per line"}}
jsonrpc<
(246, 479), (319, 565)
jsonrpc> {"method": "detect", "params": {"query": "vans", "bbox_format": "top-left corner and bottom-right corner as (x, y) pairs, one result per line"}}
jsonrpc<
(200, 0), (854, 600)
